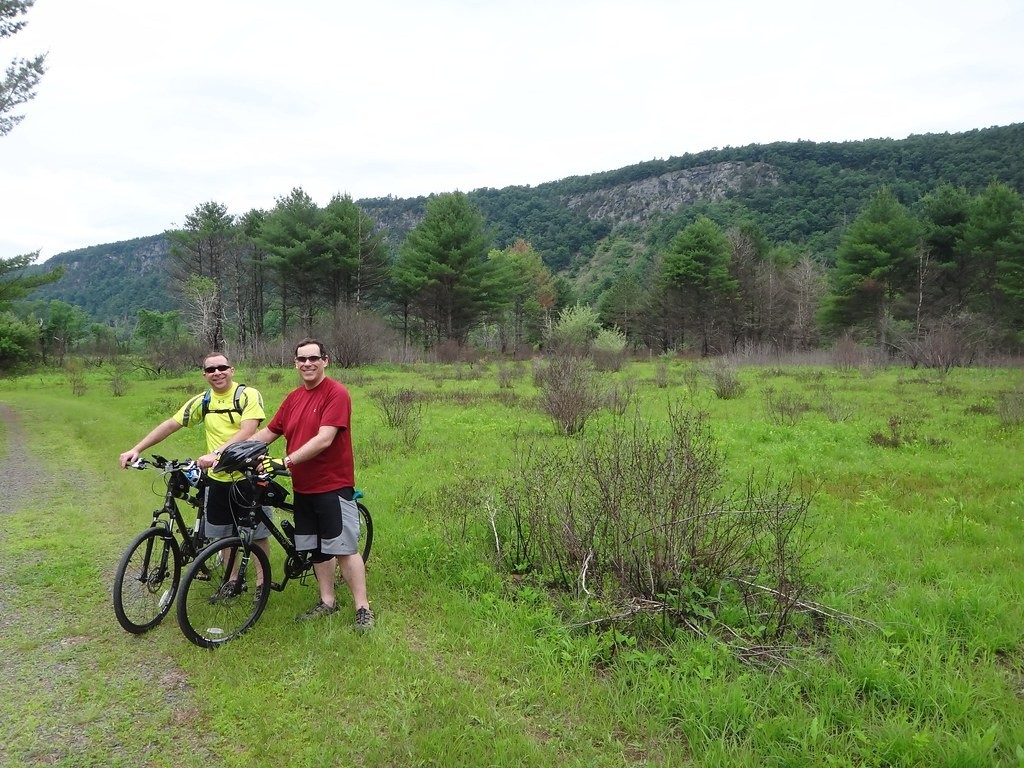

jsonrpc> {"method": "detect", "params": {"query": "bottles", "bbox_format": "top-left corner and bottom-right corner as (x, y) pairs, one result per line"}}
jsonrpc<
(281, 519), (295, 544)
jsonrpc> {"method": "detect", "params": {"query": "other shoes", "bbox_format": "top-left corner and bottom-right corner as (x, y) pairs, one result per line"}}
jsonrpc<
(211, 579), (248, 602)
(253, 583), (264, 604)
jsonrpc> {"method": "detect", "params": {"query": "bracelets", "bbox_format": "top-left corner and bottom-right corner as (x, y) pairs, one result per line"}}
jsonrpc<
(285, 456), (293, 468)
(214, 449), (220, 454)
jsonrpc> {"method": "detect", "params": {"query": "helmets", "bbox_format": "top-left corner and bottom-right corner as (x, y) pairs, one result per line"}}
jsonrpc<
(185, 467), (202, 487)
(213, 441), (269, 473)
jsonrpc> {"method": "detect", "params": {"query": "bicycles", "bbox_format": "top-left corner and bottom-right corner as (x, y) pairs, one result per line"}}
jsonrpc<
(113, 456), (373, 648)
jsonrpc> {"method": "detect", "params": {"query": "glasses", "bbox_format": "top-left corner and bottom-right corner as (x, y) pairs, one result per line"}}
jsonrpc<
(204, 365), (231, 373)
(296, 356), (326, 362)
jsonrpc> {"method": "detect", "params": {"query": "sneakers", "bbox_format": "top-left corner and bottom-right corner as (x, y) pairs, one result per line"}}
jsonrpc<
(294, 599), (339, 623)
(355, 605), (374, 625)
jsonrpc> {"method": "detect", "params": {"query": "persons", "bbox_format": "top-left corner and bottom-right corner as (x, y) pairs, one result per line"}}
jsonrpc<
(120, 351), (274, 603)
(211, 337), (374, 633)
